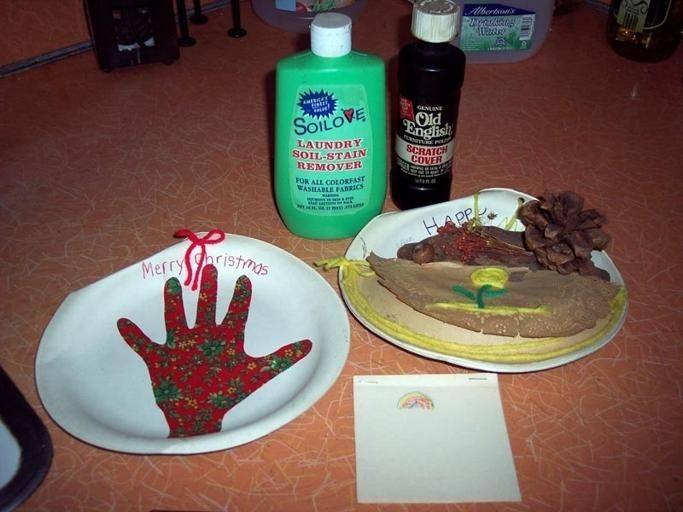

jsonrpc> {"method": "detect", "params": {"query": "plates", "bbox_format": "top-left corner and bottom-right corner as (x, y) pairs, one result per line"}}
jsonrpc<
(0, 357), (53, 512)
(35, 230), (353, 460)
(336, 188), (628, 375)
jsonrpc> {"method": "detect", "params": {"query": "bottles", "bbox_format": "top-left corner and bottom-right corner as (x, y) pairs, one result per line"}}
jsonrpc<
(384, 2), (467, 213)
(607, 0), (676, 63)
(274, 12), (388, 242)
